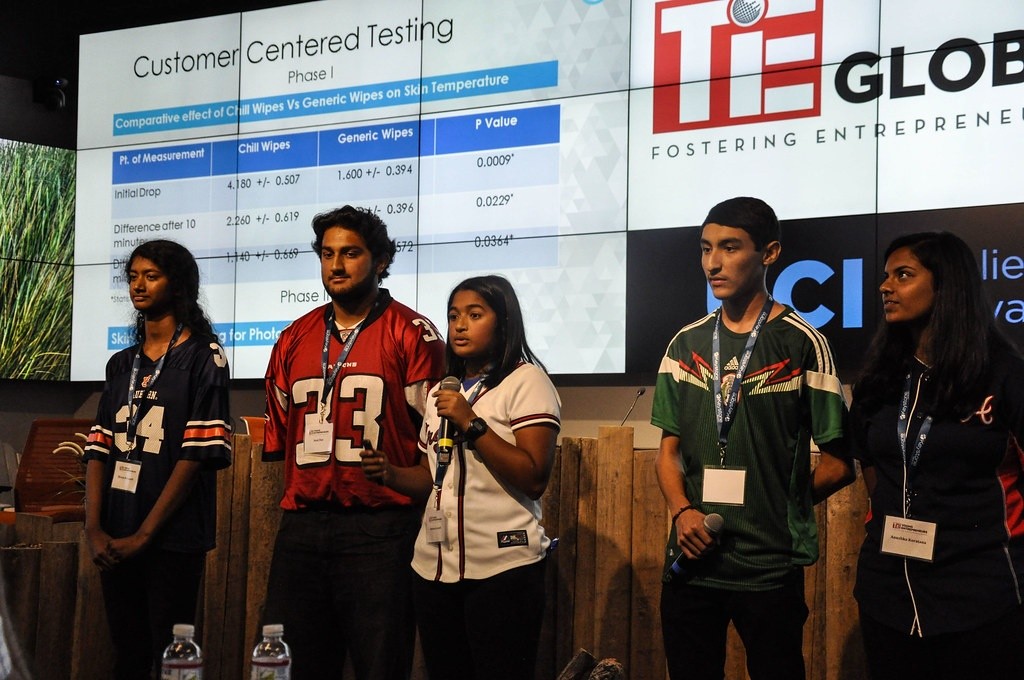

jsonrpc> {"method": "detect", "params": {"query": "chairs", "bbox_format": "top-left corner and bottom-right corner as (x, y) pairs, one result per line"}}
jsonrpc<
(13, 419), (100, 523)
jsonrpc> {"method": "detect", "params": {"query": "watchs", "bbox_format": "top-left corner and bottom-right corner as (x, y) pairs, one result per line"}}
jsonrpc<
(464, 418), (489, 443)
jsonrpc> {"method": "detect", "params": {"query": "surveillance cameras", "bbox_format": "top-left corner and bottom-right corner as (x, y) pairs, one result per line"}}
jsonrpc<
(42, 78), (76, 118)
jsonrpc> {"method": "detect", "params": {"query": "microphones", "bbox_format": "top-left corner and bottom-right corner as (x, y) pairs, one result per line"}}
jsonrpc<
(437, 376), (462, 463)
(620, 388), (646, 426)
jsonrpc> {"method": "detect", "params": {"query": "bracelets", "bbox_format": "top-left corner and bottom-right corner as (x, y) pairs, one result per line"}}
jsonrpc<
(672, 505), (700, 525)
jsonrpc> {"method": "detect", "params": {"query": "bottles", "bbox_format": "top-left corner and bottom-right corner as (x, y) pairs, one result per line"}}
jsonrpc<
(161, 624), (204, 680)
(250, 624), (292, 680)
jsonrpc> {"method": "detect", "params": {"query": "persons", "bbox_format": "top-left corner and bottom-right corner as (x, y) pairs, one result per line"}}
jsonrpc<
(359, 276), (565, 679)
(84, 241), (232, 680)
(249, 207), (452, 680)
(843, 231), (1024, 680)
(651, 196), (859, 680)
